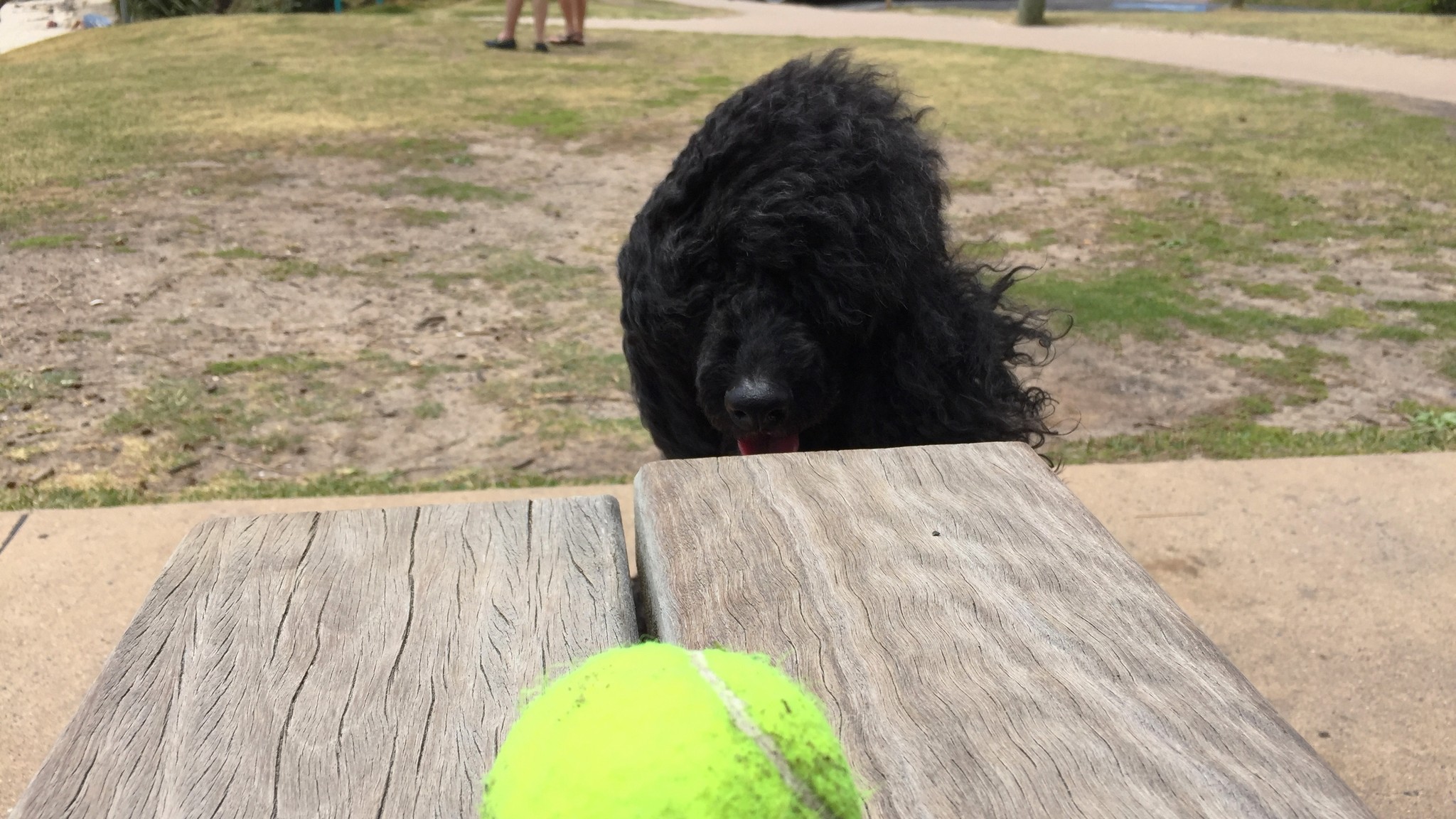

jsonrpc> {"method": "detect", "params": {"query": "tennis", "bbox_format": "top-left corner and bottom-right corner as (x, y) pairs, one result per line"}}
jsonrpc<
(483, 644), (865, 819)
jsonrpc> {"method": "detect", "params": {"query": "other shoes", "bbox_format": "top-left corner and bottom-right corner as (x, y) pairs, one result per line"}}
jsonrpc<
(482, 38), (516, 50)
(549, 32), (585, 46)
(535, 43), (548, 52)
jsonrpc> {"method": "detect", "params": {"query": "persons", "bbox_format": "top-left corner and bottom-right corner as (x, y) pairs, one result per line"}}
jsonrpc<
(483, 0), (548, 51)
(548, 0), (587, 44)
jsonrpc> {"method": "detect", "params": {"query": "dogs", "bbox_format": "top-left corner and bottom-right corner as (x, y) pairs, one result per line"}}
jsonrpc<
(615, 44), (1080, 475)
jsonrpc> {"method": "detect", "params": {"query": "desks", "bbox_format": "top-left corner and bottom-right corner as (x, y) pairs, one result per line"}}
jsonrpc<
(14, 442), (1376, 818)
